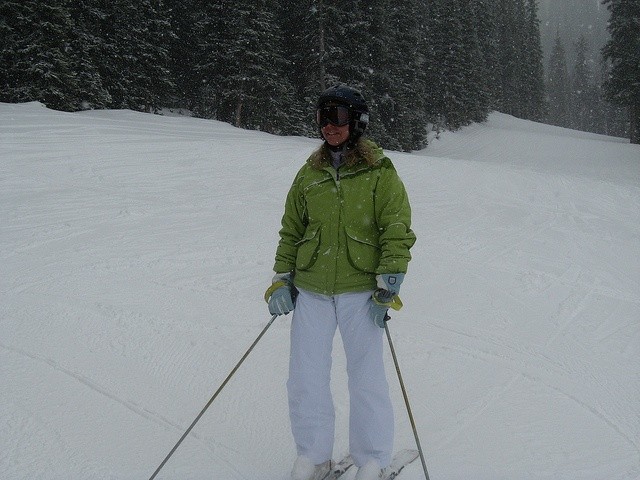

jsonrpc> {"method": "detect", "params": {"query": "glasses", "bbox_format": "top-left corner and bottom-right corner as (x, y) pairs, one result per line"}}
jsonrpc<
(317, 107), (369, 127)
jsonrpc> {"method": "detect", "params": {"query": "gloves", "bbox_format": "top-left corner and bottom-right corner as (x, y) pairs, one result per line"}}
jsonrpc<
(370, 273), (405, 328)
(264, 273), (296, 316)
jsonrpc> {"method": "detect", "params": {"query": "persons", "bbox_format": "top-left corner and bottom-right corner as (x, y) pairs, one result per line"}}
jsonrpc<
(267, 81), (416, 480)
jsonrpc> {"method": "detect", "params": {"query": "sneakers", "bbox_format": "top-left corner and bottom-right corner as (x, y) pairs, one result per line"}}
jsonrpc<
(294, 458), (336, 480)
(355, 462), (392, 480)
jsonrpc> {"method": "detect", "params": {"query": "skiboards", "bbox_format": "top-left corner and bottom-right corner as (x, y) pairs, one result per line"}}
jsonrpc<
(322, 448), (418, 480)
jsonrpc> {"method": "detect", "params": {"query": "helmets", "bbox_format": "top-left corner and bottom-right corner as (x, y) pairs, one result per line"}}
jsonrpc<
(315, 85), (370, 148)
